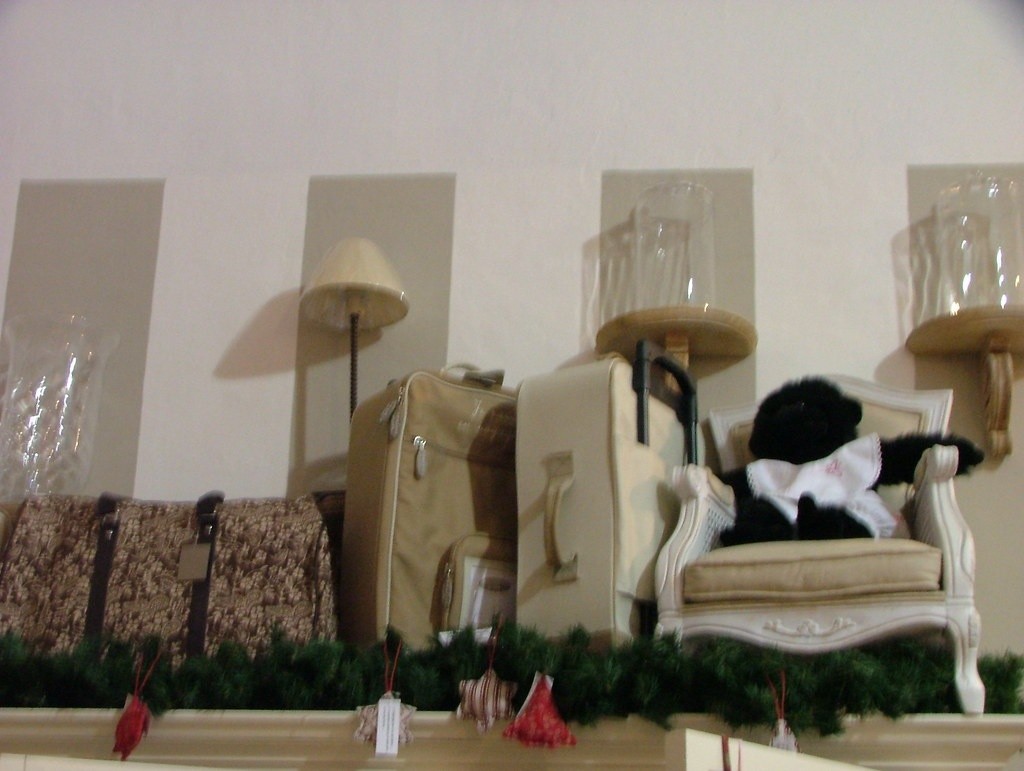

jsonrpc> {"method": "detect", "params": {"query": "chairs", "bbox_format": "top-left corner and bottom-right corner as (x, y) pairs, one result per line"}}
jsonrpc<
(653, 372), (987, 720)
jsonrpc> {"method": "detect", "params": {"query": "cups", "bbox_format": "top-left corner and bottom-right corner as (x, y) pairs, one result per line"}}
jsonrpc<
(634, 181), (715, 310)
(935, 173), (1024, 313)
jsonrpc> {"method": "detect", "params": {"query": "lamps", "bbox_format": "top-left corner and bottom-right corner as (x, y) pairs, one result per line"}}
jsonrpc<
(299, 242), (411, 421)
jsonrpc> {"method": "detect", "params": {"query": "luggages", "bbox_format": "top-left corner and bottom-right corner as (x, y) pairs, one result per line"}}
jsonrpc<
(343, 364), (521, 655)
(516, 338), (713, 655)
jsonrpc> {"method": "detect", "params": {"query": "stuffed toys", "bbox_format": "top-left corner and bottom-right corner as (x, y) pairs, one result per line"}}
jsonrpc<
(715, 376), (984, 547)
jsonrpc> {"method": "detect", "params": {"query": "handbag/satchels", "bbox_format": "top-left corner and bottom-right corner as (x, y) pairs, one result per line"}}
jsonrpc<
(0, 490), (338, 659)
